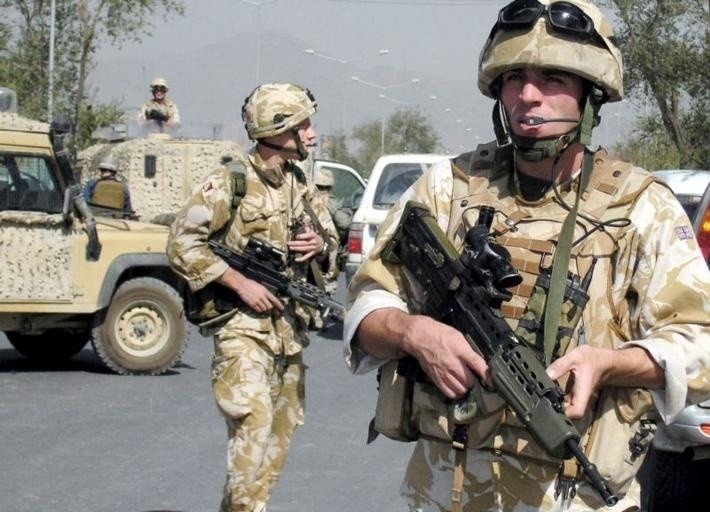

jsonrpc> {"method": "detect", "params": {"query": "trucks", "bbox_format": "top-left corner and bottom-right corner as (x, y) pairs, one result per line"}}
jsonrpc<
(310, 158), (367, 271)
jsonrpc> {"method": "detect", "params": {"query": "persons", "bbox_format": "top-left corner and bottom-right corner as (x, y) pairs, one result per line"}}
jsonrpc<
(166, 81), (340, 512)
(341, 0), (709, 512)
(306, 168), (346, 332)
(136, 78), (182, 134)
(84, 160), (133, 220)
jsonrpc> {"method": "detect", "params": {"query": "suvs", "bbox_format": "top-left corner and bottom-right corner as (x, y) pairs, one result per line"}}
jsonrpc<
(71, 139), (242, 223)
(0, 87), (192, 376)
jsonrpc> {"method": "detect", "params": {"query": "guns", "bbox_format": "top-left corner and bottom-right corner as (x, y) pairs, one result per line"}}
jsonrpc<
(208, 237), (345, 323)
(380, 199), (619, 506)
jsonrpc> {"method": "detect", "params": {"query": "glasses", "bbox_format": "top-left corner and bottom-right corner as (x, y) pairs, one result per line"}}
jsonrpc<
(488, 0), (617, 49)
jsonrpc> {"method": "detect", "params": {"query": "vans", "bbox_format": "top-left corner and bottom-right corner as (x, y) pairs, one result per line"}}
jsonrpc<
(346, 153), (450, 287)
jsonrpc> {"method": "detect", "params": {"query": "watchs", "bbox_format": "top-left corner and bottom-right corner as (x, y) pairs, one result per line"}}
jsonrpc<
(320, 243), (328, 258)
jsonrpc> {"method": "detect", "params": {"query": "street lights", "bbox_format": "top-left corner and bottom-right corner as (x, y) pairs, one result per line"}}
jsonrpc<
(304, 49), (480, 154)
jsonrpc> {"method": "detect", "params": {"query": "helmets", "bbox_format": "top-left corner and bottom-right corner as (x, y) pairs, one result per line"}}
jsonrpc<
(151, 79), (168, 91)
(241, 83), (315, 139)
(97, 156), (118, 171)
(478, 1), (624, 102)
